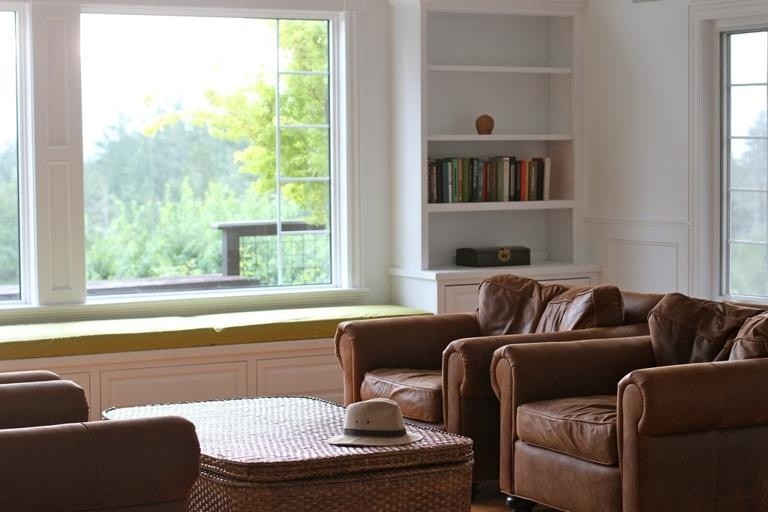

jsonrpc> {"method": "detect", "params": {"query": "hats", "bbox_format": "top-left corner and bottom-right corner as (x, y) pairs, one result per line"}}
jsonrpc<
(328, 397), (423, 447)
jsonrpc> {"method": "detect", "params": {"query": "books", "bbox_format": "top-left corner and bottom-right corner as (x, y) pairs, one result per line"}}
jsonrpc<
(427, 154), (553, 204)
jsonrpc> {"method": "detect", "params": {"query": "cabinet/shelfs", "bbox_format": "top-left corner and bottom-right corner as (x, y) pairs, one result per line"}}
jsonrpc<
(387, 0), (605, 315)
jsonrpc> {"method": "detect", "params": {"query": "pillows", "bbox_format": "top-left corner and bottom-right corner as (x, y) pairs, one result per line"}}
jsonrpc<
(470, 274), (627, 329)
(647, 294), (768, 360)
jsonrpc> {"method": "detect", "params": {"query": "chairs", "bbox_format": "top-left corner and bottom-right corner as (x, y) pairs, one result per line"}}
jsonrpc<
(490, 334), (768, 511)
(0, 369), (61, 383)
(0, 380), (202, 512)
(334, 290), (664, 504)
